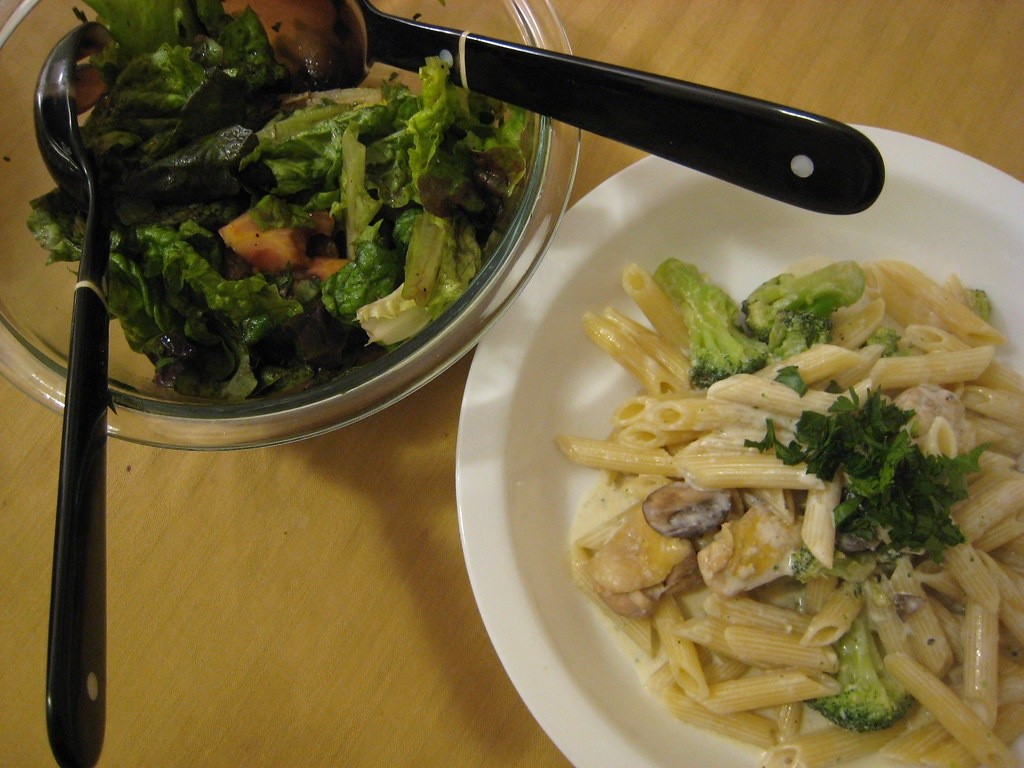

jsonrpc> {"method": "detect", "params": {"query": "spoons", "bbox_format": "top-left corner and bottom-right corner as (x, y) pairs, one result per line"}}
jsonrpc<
(34, 23), (132, 768)
(206, 0), (887, 216)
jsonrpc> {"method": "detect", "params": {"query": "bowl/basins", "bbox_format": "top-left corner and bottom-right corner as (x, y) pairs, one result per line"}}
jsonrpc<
(0, 0), (582, 451)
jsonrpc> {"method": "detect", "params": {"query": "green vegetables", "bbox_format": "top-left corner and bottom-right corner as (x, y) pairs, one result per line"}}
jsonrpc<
(740, 367), (990, 569)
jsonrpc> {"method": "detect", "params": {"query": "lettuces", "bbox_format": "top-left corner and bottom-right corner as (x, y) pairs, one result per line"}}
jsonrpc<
(28, 0), (530, 403)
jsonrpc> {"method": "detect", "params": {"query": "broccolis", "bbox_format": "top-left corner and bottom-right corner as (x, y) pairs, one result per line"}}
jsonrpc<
(801, 615), (922, 731)
(654, 261), (901, 388)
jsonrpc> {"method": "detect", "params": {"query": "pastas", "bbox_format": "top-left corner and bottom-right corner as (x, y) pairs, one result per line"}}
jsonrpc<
(551, 259), (1024, 768)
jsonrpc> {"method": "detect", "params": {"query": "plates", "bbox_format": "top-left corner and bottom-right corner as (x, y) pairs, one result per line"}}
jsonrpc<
(453, 125), (1024, 768)
